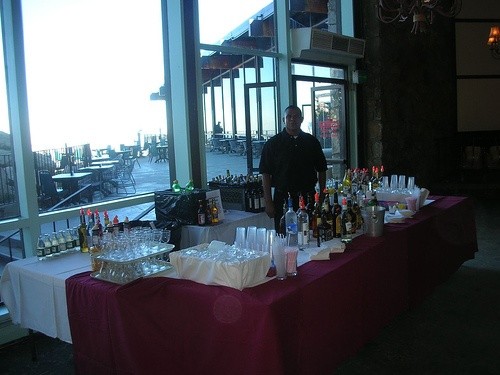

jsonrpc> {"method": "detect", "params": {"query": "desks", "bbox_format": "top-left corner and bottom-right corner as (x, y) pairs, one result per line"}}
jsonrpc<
(210, 133), (266, 157)
(0, 161), (481, 375)
(156, 145), (168, 162)
(114, 150), (129, 159)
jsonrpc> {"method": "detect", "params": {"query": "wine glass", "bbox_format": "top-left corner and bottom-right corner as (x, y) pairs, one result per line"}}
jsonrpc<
(92, 226), (170, 283)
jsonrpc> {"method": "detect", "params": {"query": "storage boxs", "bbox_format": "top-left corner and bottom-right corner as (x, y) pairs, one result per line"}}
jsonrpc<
(208, 181), (253, 211)
(155, 188), (212, 224)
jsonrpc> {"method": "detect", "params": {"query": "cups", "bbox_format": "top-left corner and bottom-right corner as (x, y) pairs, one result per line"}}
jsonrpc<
(375, 174), (430, 217)
(236, 225), (298, 280)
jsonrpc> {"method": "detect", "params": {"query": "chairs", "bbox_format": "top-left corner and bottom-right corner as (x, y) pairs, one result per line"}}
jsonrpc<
(37, 149), (171, 210)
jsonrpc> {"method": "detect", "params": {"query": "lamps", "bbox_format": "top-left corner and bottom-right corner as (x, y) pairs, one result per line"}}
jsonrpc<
(488, 26), (500, 60)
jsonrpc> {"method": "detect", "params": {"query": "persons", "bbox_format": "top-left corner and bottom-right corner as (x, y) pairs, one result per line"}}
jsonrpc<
(260, 105), (328, 238)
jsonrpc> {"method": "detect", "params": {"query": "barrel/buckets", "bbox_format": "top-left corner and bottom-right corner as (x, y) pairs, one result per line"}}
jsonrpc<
(360, 205), (386, 240)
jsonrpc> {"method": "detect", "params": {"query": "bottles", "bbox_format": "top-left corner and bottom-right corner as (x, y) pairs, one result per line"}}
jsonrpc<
(86, 211), (95, 248)
(103, 209), (109, 232)
(58, 230), (68, 254)
(43, 234), (52, 256)
(66, 228), (75, 251)
(91, 216), (102, 247)
(78, 208), (90, 253)
(171, 169), (265, 226)
(73, 227), (80, 251)
(123, 216), (131, 233)
(280, 166), (384, 245)
(37, 235), (46, 261)
(94, 209), (102, 231)
(113, 215), (119, 234)
(51, 231), (60, 255)
(107, 221), (114, 233)
